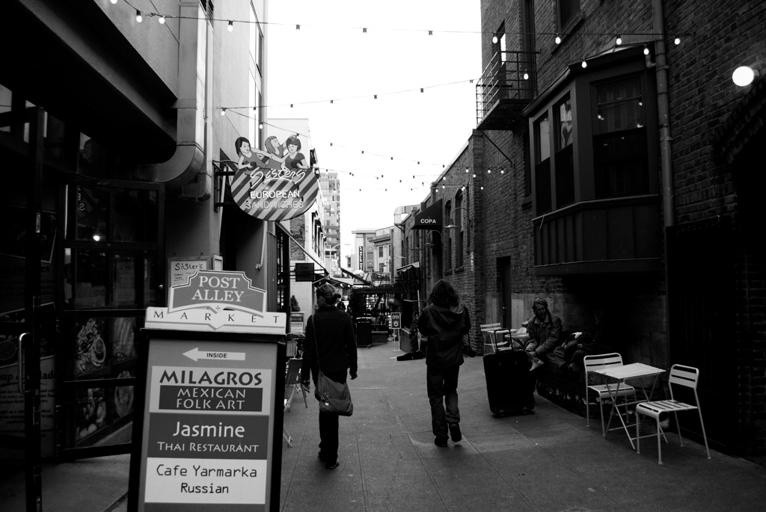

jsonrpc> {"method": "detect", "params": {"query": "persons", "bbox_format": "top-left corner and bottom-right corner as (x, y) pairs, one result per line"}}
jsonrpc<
(265, 135), (299, 171)
(298, 283), (359, 472)
(280, 136), (310, 171)
(235, 137), (272, 170)
(419, 279), (471, 451)
(525, 300), (565, 374)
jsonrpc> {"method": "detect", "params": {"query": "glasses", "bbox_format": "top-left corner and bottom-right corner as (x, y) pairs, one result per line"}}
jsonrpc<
(443, 207), (470, 230)
(423, 230), (441, 247)
(731, 65), (761, 87)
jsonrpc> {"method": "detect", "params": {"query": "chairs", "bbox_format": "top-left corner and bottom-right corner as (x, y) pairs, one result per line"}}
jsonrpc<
(317, 441), (340, 470)
(435, 422), (461, 448)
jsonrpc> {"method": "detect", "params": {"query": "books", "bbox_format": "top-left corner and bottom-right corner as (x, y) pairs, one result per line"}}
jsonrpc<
(370, 330), (389, 344)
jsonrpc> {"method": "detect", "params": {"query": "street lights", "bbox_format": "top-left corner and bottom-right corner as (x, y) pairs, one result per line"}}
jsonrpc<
(461, 342), (476, 357)
(314, 370), (354, 416)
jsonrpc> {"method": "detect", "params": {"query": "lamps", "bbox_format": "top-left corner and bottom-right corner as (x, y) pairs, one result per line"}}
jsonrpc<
(481, 322), (711, 468)
(284, 337), (309, 410)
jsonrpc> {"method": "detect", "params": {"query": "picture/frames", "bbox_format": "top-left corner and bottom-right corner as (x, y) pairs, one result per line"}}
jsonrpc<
(400, 328), (418, 353)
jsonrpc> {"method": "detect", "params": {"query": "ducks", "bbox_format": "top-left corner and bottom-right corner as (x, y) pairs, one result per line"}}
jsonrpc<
(482, 329), (537, 417)
